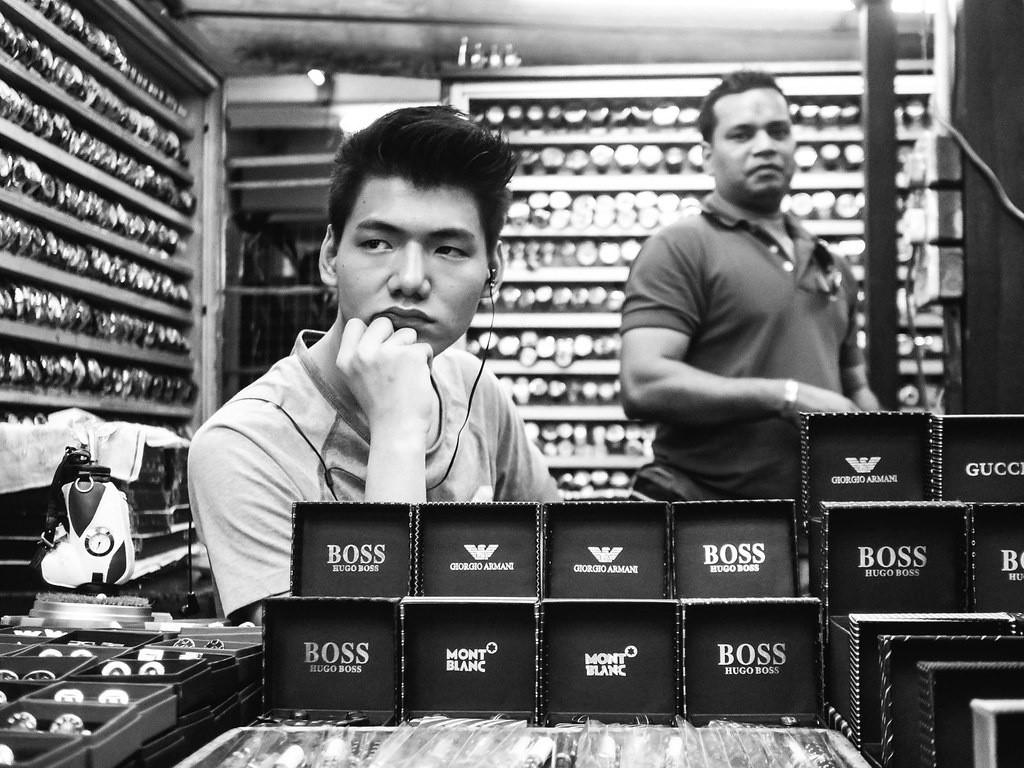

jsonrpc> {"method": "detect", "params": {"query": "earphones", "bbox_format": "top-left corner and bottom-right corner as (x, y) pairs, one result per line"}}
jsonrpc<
(489, 268), (497, 284)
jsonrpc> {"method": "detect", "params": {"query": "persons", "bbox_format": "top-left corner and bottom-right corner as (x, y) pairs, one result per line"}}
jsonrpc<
(187, 106), (562, 626)
(620, 71), (878, 501)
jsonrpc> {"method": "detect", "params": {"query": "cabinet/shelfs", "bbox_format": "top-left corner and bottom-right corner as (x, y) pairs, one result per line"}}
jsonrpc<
(0, 0), (194, 421)
(448, 74), (944, 501)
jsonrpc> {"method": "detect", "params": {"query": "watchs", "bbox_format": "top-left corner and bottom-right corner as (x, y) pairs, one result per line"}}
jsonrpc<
(0, 0), (198, 406)
(466, 103), (716, 492)
(781, 95), (946, 410)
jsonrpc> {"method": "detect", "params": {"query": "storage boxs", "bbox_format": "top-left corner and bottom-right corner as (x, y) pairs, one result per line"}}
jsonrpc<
(0, 408), (1024, 768)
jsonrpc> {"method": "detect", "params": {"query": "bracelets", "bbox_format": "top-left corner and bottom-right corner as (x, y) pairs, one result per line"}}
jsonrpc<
(779, 380), (800, 415)
